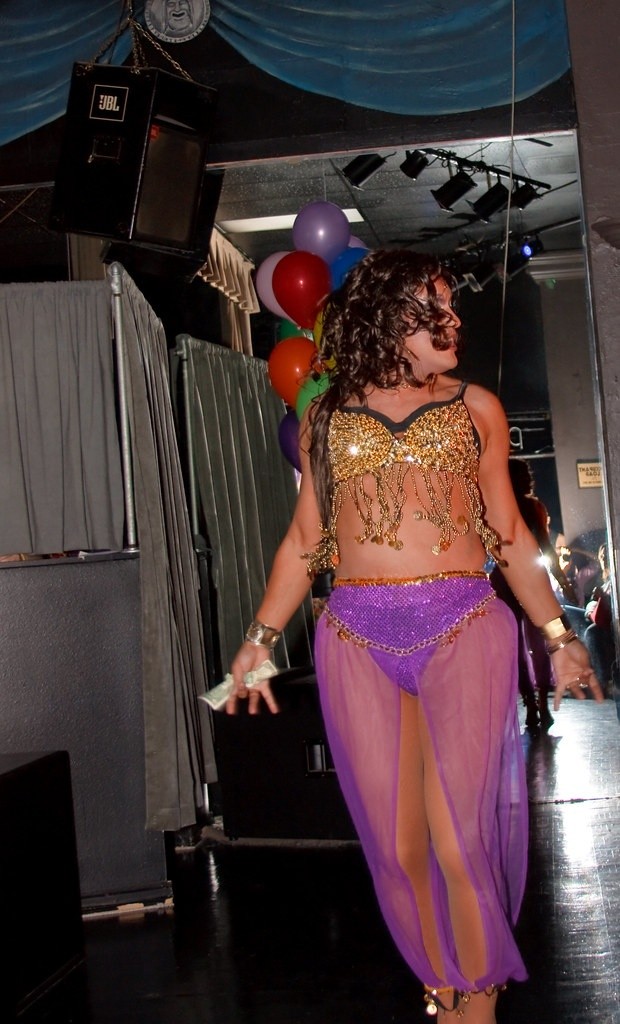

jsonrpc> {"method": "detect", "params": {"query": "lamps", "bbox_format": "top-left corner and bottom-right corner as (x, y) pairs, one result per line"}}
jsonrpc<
(341, 151), (540, 224)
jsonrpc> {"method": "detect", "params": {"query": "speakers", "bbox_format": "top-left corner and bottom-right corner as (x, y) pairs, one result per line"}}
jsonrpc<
(47, 61), (232, 263)
(0, 748), (98, 1024)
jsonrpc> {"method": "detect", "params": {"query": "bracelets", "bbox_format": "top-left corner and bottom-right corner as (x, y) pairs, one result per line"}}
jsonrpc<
(537, 613), (579, 656)
(557, 575), (569, 588)
(246, 622), (281, 651)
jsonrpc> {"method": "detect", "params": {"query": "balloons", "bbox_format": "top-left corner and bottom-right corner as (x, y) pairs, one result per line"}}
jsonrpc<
(278, 413), (302, 473)
(257, 252), (297, 325)
(331, 237), (369, 289)
(269, 337), (319, 408)
(272, 250), (332, 331)
(293, 201), (351, 264)
(296, 374), (333, 419)
(313, 304), (339, 375)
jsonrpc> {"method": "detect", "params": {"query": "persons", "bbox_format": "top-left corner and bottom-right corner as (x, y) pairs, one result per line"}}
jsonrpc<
(490, 466), (579, 726)
(225, 254), (604, 1024)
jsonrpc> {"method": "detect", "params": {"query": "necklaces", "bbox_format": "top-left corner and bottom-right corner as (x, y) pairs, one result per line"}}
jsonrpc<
(383, 380), (410, 390)
